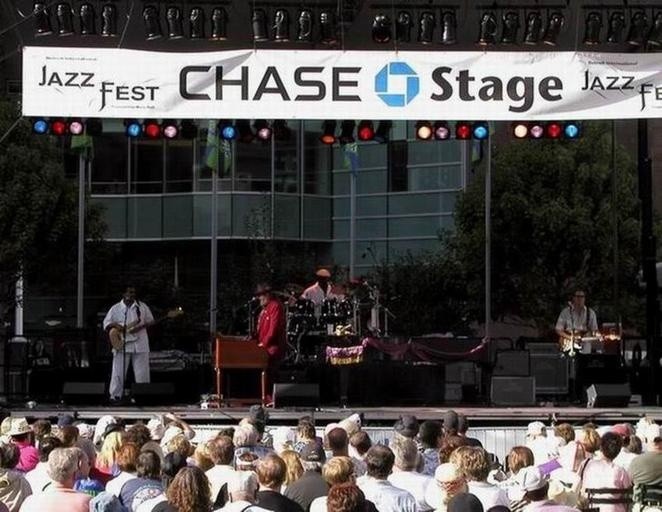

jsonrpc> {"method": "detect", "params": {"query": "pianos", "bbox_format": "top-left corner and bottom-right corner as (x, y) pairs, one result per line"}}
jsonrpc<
(213, 331), (270, 408)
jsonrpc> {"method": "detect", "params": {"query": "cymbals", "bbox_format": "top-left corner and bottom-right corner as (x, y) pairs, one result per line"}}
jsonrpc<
(272, 291), (298, 302)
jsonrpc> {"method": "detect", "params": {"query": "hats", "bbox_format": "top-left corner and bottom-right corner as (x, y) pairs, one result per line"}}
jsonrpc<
(525, 421), (545, 435)
(255, 282), (271, 294)
(300, 444), (326, 461)
(5, 417), (31, 435)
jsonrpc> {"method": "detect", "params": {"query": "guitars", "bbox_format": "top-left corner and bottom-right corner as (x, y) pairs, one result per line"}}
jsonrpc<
(558, 329), (621, 352)
(109, 307), (184, 350)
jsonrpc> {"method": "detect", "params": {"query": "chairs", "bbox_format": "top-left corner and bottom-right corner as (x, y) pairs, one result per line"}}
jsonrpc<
(640, 482), (662, 512)
(582, 483), (636, 512)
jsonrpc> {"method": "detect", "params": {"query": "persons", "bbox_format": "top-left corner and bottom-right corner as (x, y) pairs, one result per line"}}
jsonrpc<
(299, 268), (339, 326)
(103, 284), (156, 405)
(156, 302), (196, 352)
(552, 290), (601, 357)
(250, 283), (288, 409)
(0, 403), (661, 512)
(29, 338), (59, 403)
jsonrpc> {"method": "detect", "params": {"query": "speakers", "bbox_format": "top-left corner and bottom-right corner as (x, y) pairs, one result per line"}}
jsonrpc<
(272, 383), (321, 411)
(62, 382), (107, 403)
(493, 351), (530, 375)
(131, 382), (177, 406)
(585, 383), (632, 407)
(490, 376), (536, 406)
(530, 343), (569, 395)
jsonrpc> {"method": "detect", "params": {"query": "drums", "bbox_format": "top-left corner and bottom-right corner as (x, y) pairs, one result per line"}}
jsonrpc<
(341, 297), (360, 319)
(291, 300), (317, 326)
(320, 298), (346, 323)
